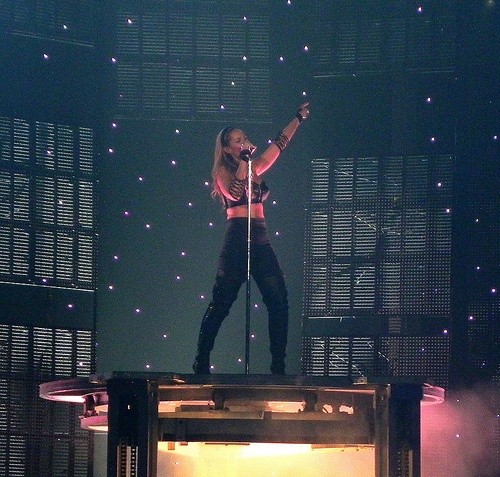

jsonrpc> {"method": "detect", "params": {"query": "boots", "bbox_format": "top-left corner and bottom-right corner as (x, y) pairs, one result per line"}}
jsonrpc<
(262, 291), (288, 375)
(193, 304), (230, 375)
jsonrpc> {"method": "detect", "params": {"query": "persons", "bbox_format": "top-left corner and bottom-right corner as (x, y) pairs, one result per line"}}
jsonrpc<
(192, 102), (310, 374)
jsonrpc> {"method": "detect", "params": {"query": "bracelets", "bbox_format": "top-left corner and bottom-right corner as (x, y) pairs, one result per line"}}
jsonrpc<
(295, 113), (305, 124)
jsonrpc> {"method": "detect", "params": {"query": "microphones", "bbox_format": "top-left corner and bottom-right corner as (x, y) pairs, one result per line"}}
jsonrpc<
(241, 145), (254, 150)
